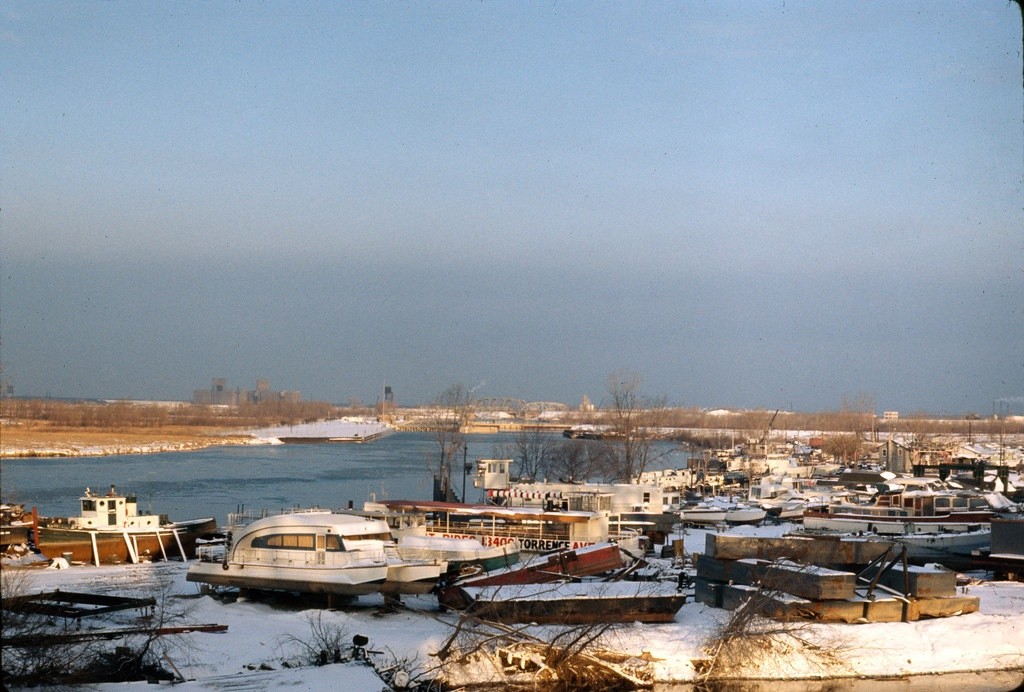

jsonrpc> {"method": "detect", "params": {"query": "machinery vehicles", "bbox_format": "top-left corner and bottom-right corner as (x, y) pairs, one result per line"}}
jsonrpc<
(759, 410), (779, 443)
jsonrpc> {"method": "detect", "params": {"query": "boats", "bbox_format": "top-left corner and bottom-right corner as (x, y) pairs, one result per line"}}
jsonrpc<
(476, 594), (686, 624)
(355, 513), (521, 582)
(0, 505), (34, 545)
(721, 502), (767, 526)
(674, 506), (728, 525)
(802, 477), (1024, 534)
(32, 484), (217, 567)
(187, 513), (448, 595)
(771, 494), (825, 521)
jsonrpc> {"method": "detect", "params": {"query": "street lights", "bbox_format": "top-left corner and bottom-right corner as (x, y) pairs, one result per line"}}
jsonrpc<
(453, 442), (467, 503)
